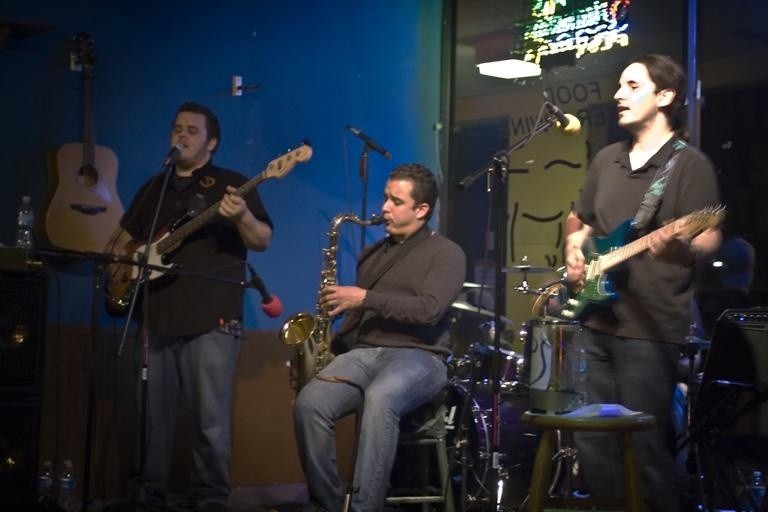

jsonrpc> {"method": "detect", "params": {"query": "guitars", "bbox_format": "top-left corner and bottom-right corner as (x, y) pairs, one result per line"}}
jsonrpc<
(41, 32), (125, 255)
(556, 207), (727, 321)
(107, 145), (312, 318)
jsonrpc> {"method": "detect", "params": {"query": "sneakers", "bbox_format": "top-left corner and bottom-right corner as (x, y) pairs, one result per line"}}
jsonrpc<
(195, 497), (230, 512)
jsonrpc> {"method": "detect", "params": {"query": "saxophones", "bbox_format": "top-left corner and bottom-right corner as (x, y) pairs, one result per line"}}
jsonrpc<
(280, 214), (384, 393)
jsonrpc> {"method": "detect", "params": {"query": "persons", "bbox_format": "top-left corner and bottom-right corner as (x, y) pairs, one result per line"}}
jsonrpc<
(292, 164), (466, 512)
(103, 104), (274, 512)
(564, 54), (723, 512)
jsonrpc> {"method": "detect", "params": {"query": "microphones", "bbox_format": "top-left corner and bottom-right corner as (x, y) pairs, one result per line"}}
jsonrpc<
(543, 99), (582, 136)
(162, 142), (185, 166)
(347, 125), (393, 161)
(248, 264), (283, 318)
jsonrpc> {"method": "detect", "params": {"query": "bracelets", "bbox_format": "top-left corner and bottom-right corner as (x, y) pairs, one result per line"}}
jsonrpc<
(681, 243), (703, 268)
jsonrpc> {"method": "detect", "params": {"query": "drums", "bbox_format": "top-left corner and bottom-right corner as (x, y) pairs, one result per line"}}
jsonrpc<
(385, 356), (538, 511)
(464, 343), (524, 382)
(522, 318), (583, 393)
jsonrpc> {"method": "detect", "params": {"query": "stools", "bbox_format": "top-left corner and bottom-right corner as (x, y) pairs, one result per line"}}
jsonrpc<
(342, 389), (455, 512)
(521, 409), (655, 512)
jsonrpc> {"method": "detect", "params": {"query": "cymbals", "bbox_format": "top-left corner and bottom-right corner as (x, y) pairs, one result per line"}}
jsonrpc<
(453, 298), (516, 326)
(463, 281), (491, 290)
(501, 264), (553, 274)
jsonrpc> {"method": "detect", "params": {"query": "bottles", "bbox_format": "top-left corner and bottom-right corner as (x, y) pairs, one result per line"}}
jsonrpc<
(58, 458), (77, 511)
(35, 459), (56, 512)
(744, 470), (766, 512)
(15, 195), (34, 249)
